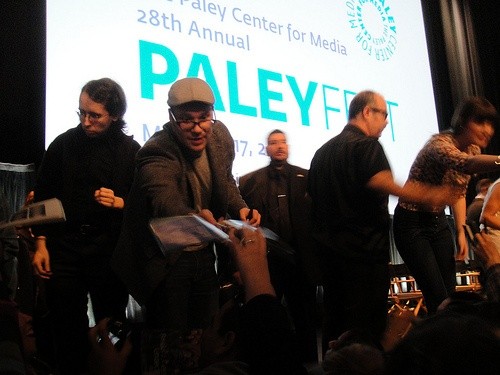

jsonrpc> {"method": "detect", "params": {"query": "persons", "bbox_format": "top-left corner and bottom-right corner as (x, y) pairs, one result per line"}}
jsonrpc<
(0, 76), (500, 375)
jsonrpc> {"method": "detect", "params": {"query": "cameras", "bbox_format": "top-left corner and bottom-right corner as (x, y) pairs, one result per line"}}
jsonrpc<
(95, 317), (136, 351)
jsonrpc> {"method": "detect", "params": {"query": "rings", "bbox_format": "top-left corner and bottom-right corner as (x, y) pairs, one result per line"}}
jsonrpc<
(242, 238), (254, 245)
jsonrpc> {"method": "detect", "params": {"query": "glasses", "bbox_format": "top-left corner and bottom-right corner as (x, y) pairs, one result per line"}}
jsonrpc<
(169, 108), (216, 129)
(370, 107), (389, 121)
(77, 111), (112, 122)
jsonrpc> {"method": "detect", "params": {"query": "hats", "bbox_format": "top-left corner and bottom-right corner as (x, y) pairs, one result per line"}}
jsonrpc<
(167, 78), (214, 110)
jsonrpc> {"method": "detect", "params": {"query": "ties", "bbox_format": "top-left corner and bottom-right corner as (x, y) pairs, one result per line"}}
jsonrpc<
(279, 175), (292, 245)
